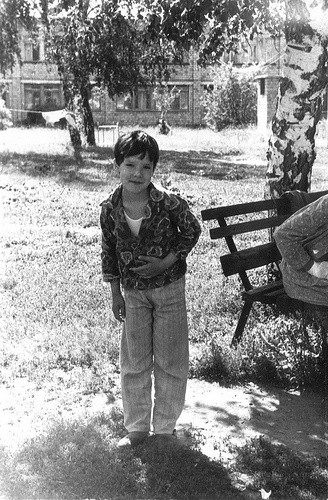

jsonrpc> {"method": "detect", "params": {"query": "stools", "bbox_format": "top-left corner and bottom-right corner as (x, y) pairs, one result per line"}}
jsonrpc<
(96, 121), (120, 145)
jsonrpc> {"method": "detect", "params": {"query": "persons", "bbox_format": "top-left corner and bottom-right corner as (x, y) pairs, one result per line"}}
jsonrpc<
(98, 129), (202, 448)
(273, 192), (328, 309)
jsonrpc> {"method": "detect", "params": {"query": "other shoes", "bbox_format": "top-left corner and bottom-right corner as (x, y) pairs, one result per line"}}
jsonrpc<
(115, 431), (178, 447)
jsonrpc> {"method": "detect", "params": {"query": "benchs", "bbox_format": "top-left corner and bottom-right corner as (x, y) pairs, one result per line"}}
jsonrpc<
(200, 189), (328, 352)
